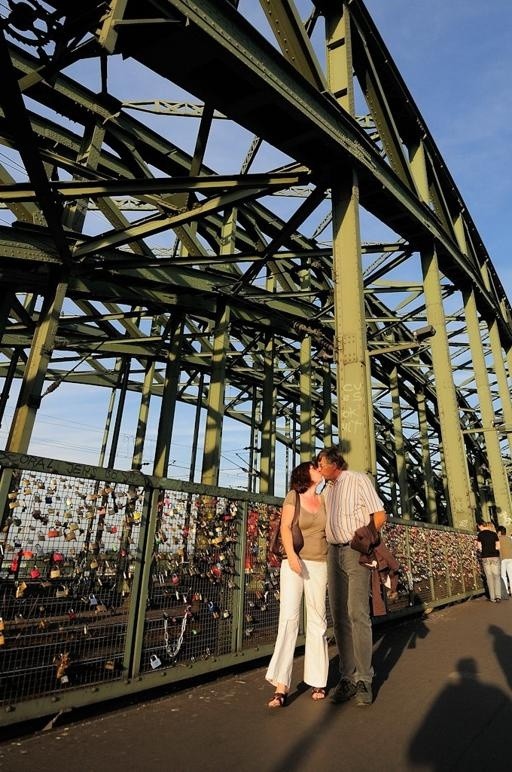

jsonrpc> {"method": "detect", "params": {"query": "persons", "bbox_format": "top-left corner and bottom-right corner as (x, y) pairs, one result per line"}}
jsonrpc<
(485, 522), (509, 600)
(496, 525), (512, 597)
(315, 447), (388, 706)
(265, 461), (331, 708)
(475, 520), (502, 603)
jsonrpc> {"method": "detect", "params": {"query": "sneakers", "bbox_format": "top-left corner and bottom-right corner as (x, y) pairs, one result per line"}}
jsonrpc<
(336, 680), (356, 697)
(488, 598), (501, 603)
(355, 672), (376, 706)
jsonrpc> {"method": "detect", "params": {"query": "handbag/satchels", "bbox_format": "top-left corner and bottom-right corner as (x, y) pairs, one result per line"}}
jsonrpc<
(272, 525), (304, 557)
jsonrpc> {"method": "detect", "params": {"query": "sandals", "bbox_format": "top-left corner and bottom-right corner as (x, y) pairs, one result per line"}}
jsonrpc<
(312, 687), (326, 700)
(268, 692), (288, 708)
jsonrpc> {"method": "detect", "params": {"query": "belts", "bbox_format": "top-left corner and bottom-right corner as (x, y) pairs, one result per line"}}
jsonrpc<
(331, 543), (349, 548)
